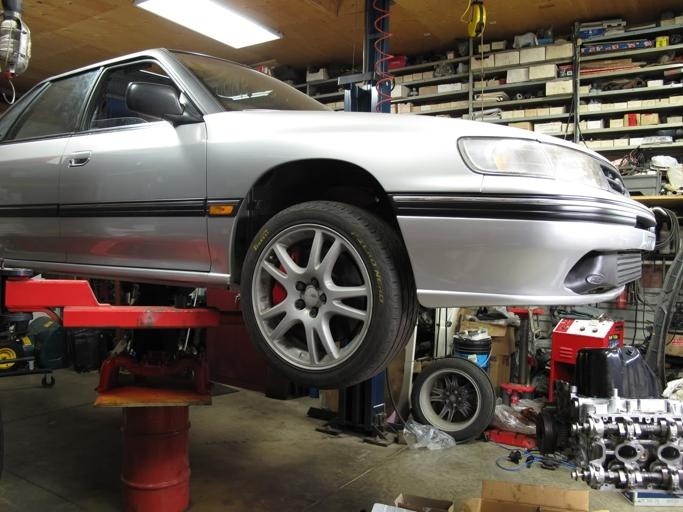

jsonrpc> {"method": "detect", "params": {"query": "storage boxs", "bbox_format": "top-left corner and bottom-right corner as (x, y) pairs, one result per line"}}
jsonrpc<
(487, 355), (512, 388)
(461, 317), (516, 356)
(392, 481), (590, 511)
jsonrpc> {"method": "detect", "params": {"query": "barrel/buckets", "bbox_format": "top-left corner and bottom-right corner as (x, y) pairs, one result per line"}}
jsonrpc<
(450, 330), (492, 370)
(120, 407), (191, 511)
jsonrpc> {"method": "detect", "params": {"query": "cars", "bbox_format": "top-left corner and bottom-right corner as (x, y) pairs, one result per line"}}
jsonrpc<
(0, 43), (668, 393)
